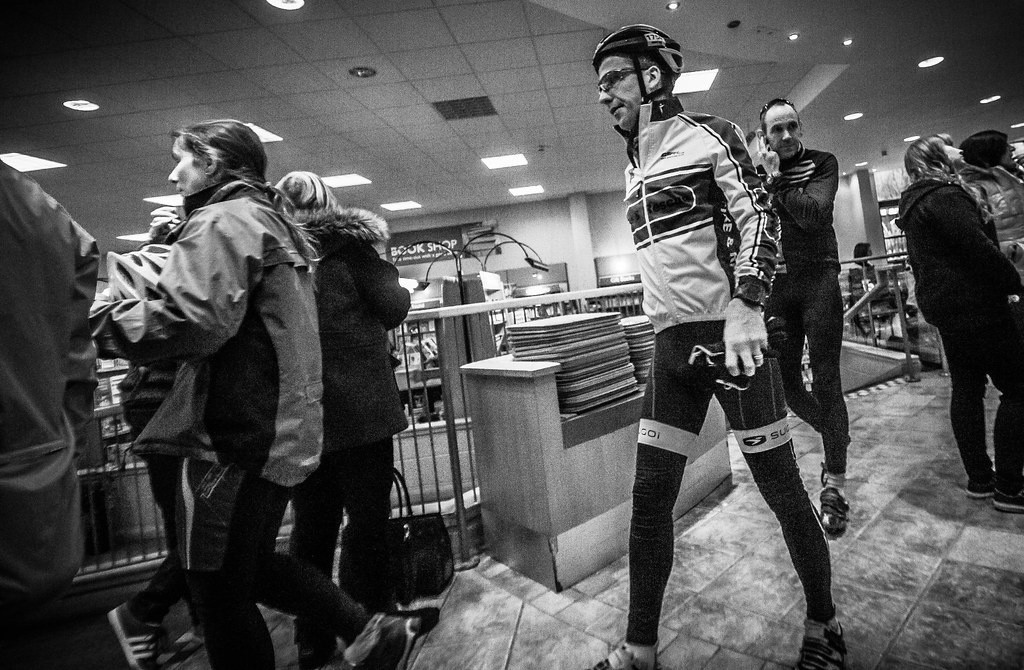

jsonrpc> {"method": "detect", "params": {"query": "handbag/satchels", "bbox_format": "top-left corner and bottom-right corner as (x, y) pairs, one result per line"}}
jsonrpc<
(339, 467), (455, 605)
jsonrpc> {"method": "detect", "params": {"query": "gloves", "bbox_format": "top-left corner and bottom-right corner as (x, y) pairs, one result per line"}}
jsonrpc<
(689, 316), (787, 393)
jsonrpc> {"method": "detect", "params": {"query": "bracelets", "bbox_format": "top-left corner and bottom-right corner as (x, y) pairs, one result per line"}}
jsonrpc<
(766, 172), (782, 185)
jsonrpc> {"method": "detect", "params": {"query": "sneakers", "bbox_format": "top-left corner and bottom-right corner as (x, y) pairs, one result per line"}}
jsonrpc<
(110, 603), (162, 670)
(819, 487), (849, 532)
(586, 641), (658, 670)
(794, 603), (848, 670)
(354, 612), (421, 668)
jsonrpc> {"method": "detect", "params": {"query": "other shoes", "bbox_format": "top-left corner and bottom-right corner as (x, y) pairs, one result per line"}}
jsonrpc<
(990, 488), (1024, 512)
(965, 480), (994, 497)
(293, 618), (335, 670)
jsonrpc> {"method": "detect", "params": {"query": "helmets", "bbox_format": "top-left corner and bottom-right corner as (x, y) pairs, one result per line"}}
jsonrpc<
(593, 23), (683, 81)
(105, 242), (172, 299)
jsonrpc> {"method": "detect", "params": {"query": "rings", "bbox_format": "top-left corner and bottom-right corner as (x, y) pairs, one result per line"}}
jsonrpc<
(753, 352), (763, 360)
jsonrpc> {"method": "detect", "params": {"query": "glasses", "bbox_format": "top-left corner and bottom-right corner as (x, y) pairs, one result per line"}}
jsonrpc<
(596, 64), (649, 94)
(758, 98), (794, 119)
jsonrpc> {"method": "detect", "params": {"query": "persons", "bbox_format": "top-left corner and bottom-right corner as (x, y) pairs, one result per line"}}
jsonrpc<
(848, 242), (918, 335)
(275, 172), (410, 670)
(88, 119), (420, 670)
(592, 25), (846, 670)
(894, 134), (1024, 512)
(957, 129), (1024, 286)
(755, 98), (852, 535)
(0, 159), (100, 670)
(106, 362), (187, 670)
